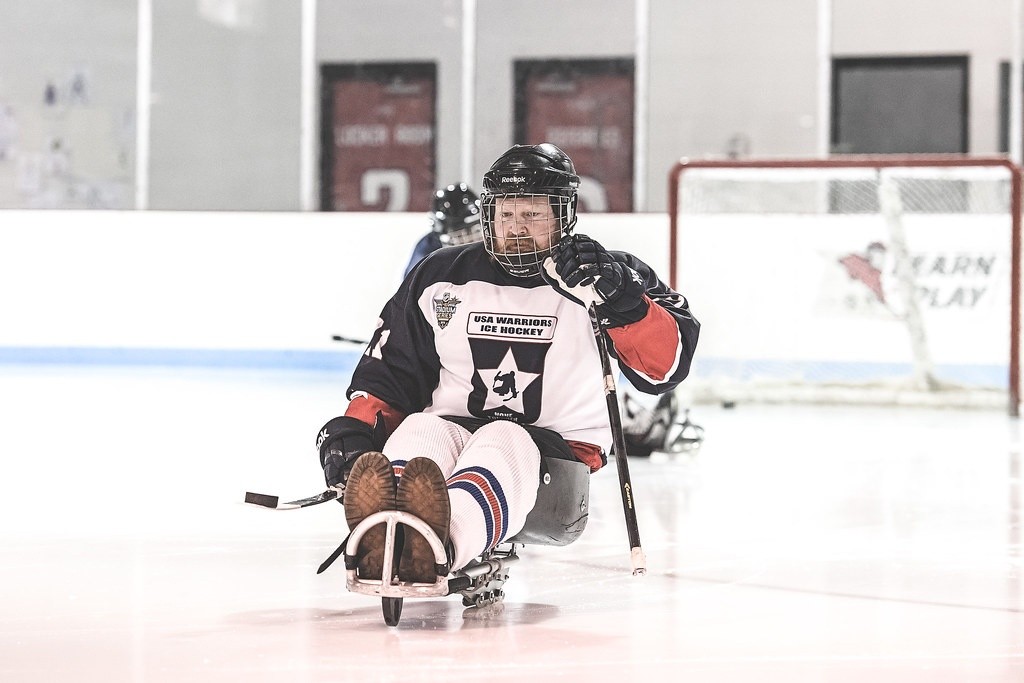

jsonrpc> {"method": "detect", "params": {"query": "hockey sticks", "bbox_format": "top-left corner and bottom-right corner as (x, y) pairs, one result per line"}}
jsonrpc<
(242, 482), (343, 512)
(542, 254), (649, 576)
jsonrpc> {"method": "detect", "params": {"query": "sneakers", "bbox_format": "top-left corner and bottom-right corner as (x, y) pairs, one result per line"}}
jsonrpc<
(343, 452), (450, 583)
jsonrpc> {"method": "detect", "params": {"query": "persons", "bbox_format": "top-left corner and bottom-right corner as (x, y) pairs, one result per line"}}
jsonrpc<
(831, 241), (888, 309)
(316, 143), (701, 583)
(405, 181), (482, 282)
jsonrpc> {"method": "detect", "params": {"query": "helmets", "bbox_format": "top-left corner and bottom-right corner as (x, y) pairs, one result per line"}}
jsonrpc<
(481, 143), (580, 278)
(423, 183), (481, 254)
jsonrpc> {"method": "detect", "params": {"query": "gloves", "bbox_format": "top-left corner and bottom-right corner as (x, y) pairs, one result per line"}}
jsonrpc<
(537, 230), (645, 323)
(314, 408), (390, 507)
